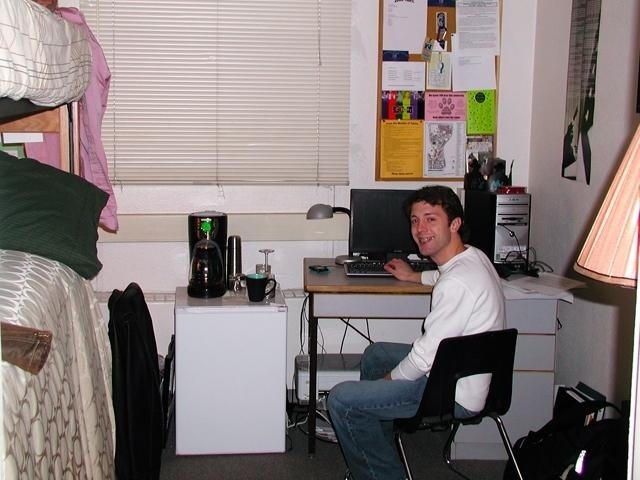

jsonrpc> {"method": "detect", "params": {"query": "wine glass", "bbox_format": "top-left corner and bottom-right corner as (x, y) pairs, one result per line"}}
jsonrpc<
(258, 249), (275, 299)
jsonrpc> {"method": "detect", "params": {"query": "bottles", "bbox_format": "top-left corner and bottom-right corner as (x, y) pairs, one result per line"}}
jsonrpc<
(227, 234), (241, 291)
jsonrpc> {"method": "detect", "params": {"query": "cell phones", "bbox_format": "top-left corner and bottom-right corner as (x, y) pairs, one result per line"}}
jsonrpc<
(308, 265), (329, 272)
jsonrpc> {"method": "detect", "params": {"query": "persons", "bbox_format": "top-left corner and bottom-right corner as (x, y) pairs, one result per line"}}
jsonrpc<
(327, 186), (508, 480)
(381, 91), (425, 119)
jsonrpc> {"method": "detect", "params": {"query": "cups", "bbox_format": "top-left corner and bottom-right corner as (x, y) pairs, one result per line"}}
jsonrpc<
(245, 273), (276, 303)
(256, 264), (271, 274)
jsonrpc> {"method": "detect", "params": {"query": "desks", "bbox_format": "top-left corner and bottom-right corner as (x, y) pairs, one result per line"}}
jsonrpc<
(303, 257), (559, 461)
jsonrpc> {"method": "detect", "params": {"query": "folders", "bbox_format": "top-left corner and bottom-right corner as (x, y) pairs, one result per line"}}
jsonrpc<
(553, 386), (598, 428)
(575, 381), (606, 422)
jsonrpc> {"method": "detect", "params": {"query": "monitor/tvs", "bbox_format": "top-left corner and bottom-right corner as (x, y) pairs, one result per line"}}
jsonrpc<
(349, 189), (420, 261)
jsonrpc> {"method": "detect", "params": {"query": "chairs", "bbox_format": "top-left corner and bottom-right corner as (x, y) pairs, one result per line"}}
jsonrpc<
(398, 328), (524, 480)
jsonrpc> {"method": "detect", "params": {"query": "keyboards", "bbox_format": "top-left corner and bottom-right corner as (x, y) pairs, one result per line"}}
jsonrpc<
(344, 260), (438, 276)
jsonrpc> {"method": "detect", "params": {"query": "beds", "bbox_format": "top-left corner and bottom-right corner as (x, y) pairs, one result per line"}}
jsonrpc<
(2, 0), (112, 480)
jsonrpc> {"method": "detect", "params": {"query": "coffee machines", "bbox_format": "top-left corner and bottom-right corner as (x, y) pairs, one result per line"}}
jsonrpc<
(187, 210), (228, 299)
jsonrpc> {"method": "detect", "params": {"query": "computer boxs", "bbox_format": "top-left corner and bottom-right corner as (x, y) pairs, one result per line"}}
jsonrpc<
(464, 189), (531, 274)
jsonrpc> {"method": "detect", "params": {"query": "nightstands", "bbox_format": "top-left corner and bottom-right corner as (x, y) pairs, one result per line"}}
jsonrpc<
(173, 286), (289, 456)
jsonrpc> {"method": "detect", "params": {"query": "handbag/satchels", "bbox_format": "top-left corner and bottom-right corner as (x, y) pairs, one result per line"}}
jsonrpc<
(503, 400), (625, 479)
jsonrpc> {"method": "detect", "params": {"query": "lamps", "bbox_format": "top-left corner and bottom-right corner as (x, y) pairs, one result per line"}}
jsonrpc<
(306, 203), (361, 265)
(573, 120), (640, 290)
